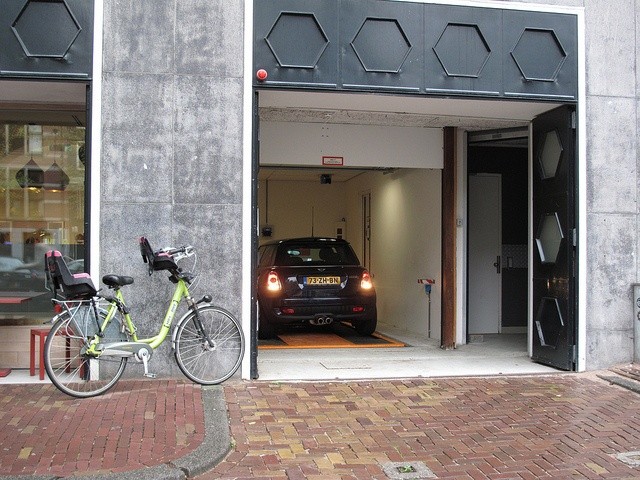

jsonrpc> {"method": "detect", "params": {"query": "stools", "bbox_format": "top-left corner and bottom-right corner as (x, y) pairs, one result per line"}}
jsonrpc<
(31, 328), (85, 380)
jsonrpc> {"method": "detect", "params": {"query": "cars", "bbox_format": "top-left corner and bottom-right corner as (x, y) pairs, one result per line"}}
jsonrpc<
(257, 237), (377, 339)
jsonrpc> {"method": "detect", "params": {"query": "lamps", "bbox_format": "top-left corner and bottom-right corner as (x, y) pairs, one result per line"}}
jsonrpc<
(16, 150), (43, 192)
(42, 131), (70, 193)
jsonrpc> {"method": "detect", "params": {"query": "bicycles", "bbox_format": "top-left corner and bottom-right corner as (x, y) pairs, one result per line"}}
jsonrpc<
(43, 236), (244, 397)
